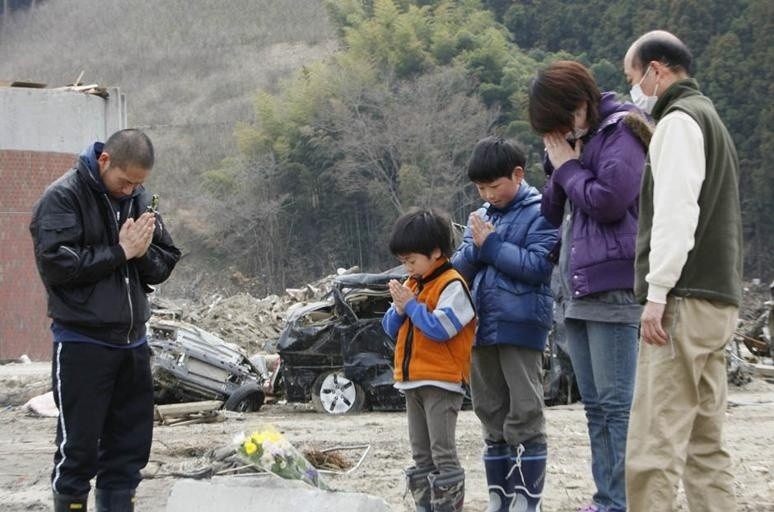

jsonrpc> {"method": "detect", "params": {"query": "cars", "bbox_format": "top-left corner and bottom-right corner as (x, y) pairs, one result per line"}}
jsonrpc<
(274, 261), (582, 415)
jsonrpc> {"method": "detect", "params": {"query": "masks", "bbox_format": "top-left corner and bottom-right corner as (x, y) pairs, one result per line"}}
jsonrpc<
(564, 112), (589, 140)
(629, 64), (660, 114)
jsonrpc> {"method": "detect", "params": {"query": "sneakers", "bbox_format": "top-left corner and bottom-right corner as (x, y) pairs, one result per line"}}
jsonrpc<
(577, 505), (613, 512)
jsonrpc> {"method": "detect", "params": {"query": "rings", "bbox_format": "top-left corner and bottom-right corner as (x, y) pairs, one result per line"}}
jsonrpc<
(552, 143), (557, 147)
(546, 143), (552, 149)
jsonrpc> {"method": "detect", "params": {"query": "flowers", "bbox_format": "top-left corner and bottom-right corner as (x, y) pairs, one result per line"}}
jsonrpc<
(230, 415), (334, 493)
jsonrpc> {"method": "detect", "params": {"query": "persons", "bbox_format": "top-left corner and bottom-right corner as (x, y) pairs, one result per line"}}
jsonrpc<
(446, 135), (567, 512)
(526, 58), (648, 511)
(380, 208), (478, 512)
(28, 128), (181, 511)
(620, 30), (745, 512)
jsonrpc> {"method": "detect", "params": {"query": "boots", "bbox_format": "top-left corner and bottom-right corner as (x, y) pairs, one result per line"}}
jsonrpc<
(427, 467), (465, 512)
(482, 454), (510, 512)
(505, 455), (547, 512)
(94, 487), (135, 512)
(52, 491), (89, 512)
(406, 465), (436, 512)
